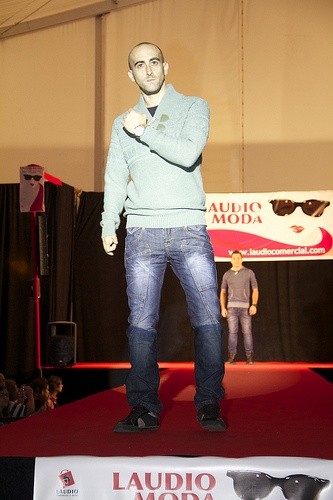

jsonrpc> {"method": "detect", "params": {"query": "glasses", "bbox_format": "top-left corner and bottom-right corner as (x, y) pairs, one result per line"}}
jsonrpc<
(226, 469), (330, 500)
(269, 198), (330, 217)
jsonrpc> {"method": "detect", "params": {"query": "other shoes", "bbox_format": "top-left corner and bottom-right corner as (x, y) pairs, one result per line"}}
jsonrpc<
(245, 353), (256, 365)
(196, 404), (229, 432)
(225, 353), (238, 365)
(113, 400), (161, 432)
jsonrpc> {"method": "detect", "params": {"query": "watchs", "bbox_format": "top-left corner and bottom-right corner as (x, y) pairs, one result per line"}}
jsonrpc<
(132, 123), (149, 138)
(252, 304), (257, 308)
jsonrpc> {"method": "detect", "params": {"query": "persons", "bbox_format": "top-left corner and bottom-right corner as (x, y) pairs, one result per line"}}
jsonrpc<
(0, 373), (65, 426)
(219, 250), (259, 365)
(98, 40), (227, 434)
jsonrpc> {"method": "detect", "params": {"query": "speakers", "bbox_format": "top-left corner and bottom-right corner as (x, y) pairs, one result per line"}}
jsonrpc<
(45, 336), (78, 365)
